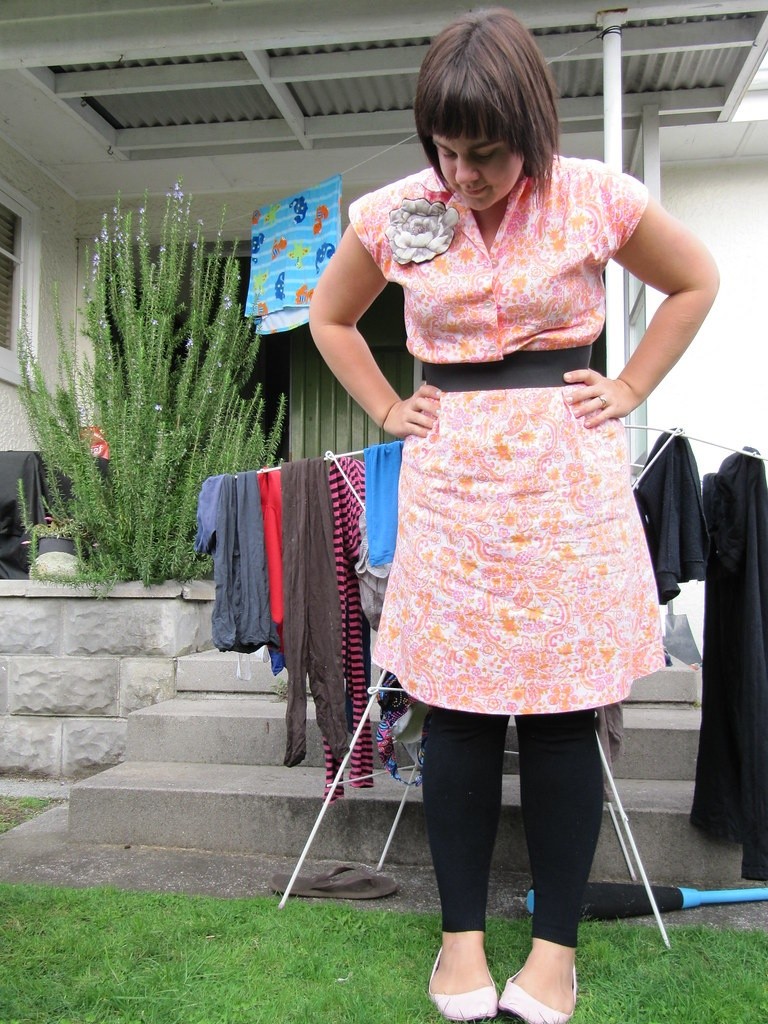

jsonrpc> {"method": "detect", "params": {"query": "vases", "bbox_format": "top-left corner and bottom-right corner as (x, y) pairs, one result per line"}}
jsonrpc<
(38, 535), (74, 555)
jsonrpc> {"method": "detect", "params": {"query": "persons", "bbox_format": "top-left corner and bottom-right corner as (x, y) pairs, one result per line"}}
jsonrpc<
(309, 8), (721, 1024)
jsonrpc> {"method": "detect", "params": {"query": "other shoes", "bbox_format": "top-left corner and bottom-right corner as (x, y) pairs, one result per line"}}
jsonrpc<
(428, 943), (498, 1021)
(497, 955), (577, 1024)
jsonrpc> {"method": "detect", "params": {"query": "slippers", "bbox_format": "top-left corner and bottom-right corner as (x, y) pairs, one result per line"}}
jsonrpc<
(270, 862), (400, 898)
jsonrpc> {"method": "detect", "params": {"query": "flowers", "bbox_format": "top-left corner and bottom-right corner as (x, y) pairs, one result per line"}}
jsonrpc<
(20, 516), (88, 545)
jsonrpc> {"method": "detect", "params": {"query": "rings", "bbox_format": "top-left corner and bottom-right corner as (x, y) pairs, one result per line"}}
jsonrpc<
(598, 395), (609, 408)
